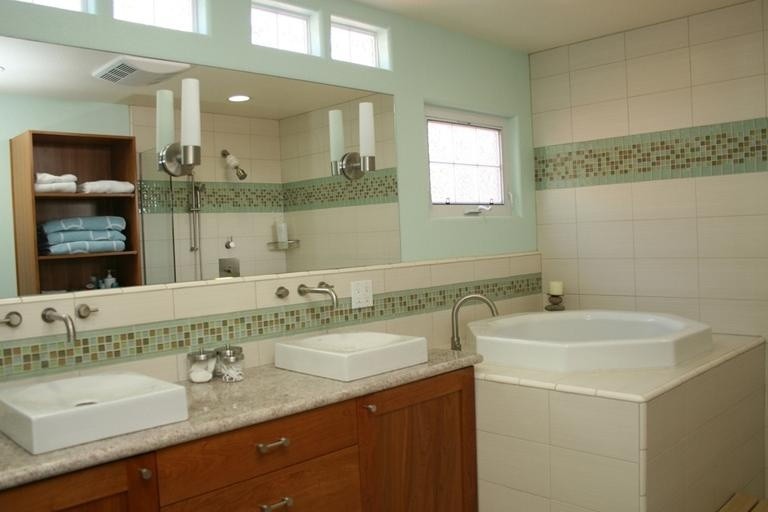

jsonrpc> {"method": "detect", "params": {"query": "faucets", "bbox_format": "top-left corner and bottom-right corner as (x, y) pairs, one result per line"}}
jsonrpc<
(297, 284), (339, 311)
(41, 307), (76, 344)
(450, 294), (499, 351)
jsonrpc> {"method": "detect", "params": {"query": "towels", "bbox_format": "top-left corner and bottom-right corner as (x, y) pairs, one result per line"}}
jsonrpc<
(40, 214), (126, 255)
(35, 173), (77, 184)
(80, 180), (134, 194)
(34, 181), (77, 194)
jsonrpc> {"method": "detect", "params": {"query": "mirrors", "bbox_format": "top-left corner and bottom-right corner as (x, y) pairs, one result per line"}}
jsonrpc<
(0, 38), (401, 300)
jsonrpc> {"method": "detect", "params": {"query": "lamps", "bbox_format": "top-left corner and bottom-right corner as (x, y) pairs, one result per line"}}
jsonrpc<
(220, 147), (248, 181)
(344, 101), (377, 180)
(156, 92), (176, 176)
(327, 107), (350, 179)
(163, 78), (201, 179)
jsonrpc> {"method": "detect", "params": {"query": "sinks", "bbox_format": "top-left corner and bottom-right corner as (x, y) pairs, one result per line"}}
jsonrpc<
(274, 331), (429, 383)
(0, 367), (189, 455)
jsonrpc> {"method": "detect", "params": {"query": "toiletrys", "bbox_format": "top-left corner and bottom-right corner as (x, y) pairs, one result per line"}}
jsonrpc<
(104, 269), (117, 288)
(276, 216), (289, 249)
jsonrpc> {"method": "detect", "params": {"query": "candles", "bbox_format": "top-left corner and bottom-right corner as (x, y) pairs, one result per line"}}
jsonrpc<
(548, 280), (563, 295)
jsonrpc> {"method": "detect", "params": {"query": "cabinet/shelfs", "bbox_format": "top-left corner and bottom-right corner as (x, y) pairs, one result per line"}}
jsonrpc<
(359, 367), (479, 511)
(155, 399), (363, 509)
(0, 456), (158, 510)
(10, 130), (143, 293)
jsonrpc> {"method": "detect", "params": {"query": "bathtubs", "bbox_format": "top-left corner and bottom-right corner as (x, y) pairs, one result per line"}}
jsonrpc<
(466, 311), (712, 370)
